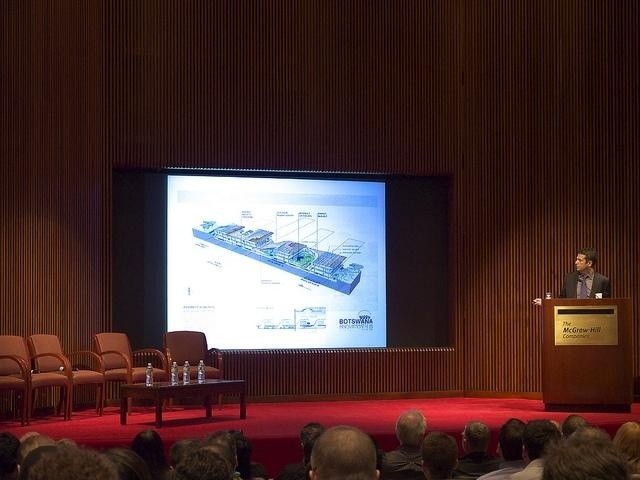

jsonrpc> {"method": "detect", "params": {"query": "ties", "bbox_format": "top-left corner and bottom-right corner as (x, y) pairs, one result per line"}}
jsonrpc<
(579, 275), (588, 297)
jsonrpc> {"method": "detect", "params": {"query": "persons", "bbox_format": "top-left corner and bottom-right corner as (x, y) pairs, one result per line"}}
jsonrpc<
(532, 248), (611, 308)
(274, 409), (639, 479)
(1, 428), (267, 479)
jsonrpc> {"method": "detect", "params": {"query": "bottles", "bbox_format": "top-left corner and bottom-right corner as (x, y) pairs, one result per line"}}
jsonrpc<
(197, 360), (205, 384)
(145, 363), (154, 386)
(183, 361), (190, 384)
(170, 361), (179, 384)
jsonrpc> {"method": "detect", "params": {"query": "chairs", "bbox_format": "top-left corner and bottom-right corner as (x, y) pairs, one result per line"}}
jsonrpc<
(26, 333), (107, 422)
(160, 330), (224, 412)
(0, 354), (28, 427)
(92, 332), (169, 417)
(0, 334), (69, 423)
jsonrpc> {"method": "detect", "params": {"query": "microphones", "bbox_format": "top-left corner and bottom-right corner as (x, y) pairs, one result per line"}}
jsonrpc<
(577, 278), (600, 301)
